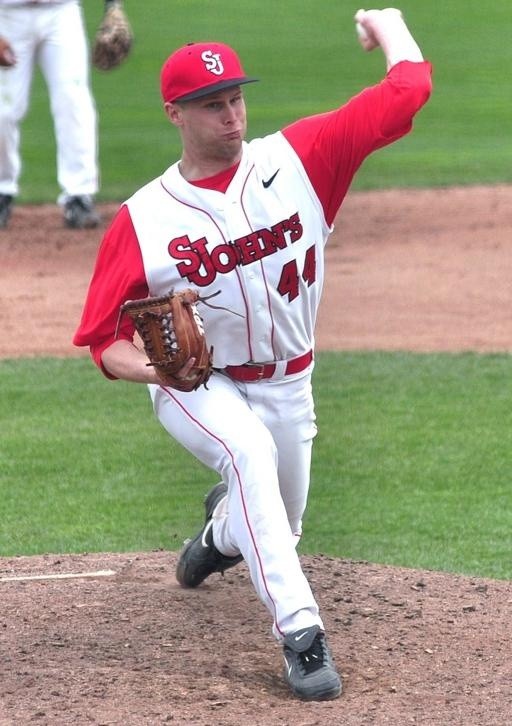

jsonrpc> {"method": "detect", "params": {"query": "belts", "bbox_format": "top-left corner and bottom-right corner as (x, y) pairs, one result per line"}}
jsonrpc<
(222, 348), (314, 383)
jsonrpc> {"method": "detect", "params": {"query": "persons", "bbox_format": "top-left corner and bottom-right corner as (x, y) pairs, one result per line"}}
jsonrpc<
(70, 7), (436, 701)
(0, 1), (133, 230)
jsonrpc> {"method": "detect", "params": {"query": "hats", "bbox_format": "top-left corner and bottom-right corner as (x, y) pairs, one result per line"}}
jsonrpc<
(159, 41), (262, 105)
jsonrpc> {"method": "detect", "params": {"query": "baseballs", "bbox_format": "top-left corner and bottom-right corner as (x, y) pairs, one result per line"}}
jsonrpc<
(356, 9), (384, 40)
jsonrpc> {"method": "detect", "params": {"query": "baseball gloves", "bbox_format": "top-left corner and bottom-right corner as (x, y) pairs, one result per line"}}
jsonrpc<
(93, 0), (134, 68)
(123, 289), (213, 392)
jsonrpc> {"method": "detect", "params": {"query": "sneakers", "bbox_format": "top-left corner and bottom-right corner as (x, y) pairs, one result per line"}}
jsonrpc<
(62, 195), (103, 231)
(0, 193), (15, 231)
(174, 480), (246, 589)
(280, 623), (344, 703)
